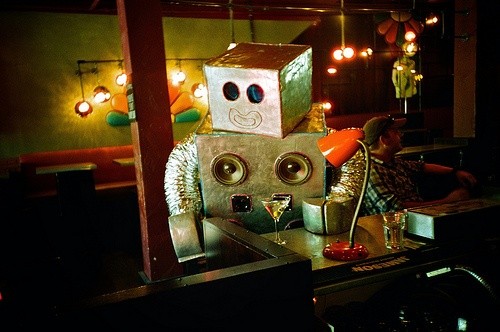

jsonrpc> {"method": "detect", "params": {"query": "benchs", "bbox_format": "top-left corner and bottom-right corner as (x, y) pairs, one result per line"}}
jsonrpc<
(0, 145), (137, 204)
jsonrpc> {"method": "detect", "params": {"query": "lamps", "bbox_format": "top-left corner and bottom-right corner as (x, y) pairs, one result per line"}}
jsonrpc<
(318, 126), (371, 261)
(115, 62), (127, 87)
(333, 0), (355, 60)
(74, 63), (94, 116)
(91, 64), (111, 104)
(172, 60), (206, 98)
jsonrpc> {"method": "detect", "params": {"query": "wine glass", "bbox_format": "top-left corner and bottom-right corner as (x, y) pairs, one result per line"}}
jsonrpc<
(258, 196), (291, 245)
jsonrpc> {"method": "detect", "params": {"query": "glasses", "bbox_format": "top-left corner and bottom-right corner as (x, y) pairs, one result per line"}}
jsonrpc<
(375, 113), (394, 137)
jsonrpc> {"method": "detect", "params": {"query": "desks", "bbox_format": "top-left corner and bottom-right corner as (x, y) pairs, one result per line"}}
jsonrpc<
(34, 161), (106, 263)
(259, 212), (500, 298)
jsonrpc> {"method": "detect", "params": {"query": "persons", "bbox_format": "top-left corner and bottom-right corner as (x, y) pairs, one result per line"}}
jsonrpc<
(363, 115), (477, 216)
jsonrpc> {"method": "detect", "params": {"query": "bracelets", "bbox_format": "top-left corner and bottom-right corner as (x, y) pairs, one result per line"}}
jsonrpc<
(450, 168), (459, 175)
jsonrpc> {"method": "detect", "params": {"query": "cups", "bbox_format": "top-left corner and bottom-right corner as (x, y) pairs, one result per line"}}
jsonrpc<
(381, 211), (408, 251)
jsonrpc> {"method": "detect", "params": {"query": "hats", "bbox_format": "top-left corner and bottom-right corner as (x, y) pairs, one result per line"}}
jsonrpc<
(361, 116), (407, 146)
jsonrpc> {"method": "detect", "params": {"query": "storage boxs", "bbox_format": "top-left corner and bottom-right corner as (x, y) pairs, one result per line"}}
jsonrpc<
(402, 197), (500, 239)
